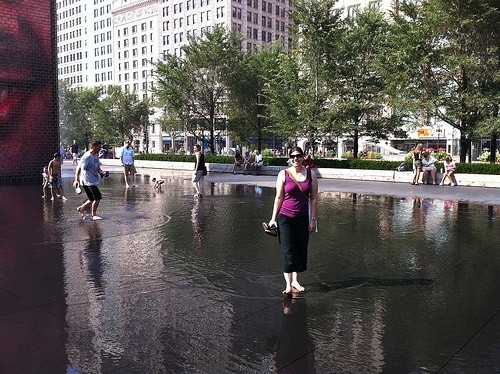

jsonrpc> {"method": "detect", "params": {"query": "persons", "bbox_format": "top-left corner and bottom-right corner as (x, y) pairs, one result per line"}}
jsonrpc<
(232, 150), (242, 174)
(192, 144), (205, 198)
(120, 140), (137, 187)
(103, 142), (109, 159)
(252, 150), (263, 175)
(411, 144), (423, 185)
(60, 142), (66, 170)
(70, 140), (78, 164)
(243, 151), (251, 175)
(422, 152), (438, 184)
(74, 140), (105, 220)
(440, 157), (456, 186)
(269, 147), (318, 293)
(272, 149), (282, 156)
(42, 166), (61, 197)
(48, 153), (67, 200)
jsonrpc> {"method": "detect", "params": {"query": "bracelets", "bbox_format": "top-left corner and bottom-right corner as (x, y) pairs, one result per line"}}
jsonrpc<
(311, 216), (317, 220)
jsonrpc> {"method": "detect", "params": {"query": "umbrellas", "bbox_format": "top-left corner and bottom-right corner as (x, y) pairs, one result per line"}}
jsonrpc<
(423, 147), (446, 152)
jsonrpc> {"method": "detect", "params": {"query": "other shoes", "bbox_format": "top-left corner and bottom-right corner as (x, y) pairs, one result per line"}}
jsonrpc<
(262, 222), (278, 237)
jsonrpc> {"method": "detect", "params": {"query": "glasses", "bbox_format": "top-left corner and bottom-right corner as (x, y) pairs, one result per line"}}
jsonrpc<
(290, 154), (302, 158)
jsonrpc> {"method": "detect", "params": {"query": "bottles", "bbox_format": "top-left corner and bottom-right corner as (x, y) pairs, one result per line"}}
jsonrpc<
(440, 168), (443, 173)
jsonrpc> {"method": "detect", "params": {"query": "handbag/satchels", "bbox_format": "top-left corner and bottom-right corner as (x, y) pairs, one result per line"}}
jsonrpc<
(203, 166), (207, 175)
